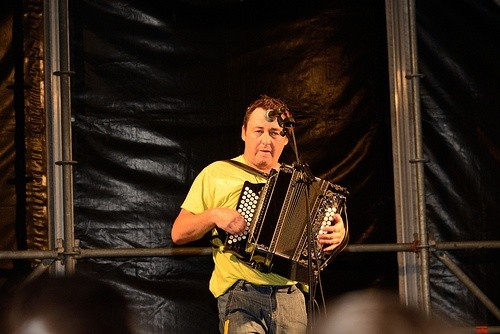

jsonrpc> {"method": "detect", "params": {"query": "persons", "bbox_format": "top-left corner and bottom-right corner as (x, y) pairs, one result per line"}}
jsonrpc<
(0, 271), (477, 334)
(171, 94), (349, 334)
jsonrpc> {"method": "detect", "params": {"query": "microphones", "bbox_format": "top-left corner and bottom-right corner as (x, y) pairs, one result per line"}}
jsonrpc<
(265, 106), (286, 122)
